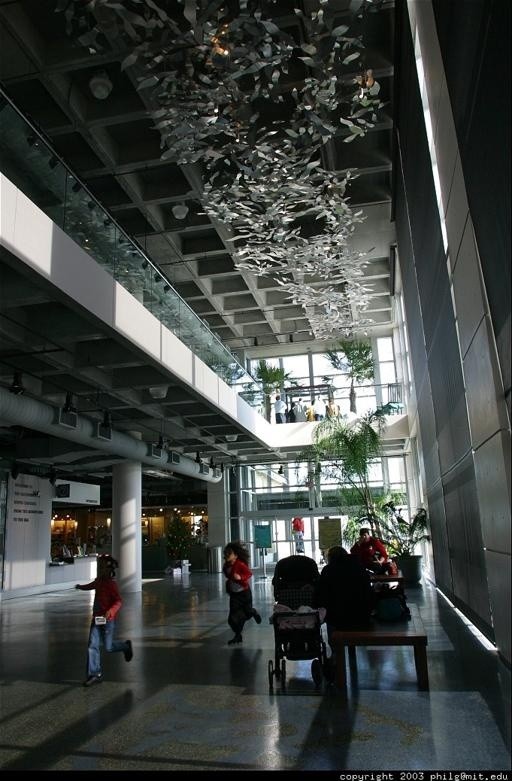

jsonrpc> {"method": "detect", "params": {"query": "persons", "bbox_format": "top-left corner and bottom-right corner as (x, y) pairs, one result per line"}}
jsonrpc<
(291, 515), (306, 552)
(272, 394), (343, 423)
(321, 544), (378, 664)
(74, 554), (134, 685)
(350, 526), (388, 567)
(222, 538), (262, 646)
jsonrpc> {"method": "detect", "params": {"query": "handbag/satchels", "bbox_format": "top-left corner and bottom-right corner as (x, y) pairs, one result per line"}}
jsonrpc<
(373, 583), (410, 620)
(388, 562), (397, 575)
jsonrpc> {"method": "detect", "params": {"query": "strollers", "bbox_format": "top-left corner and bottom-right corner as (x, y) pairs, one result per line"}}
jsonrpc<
(265, 554), (331, 691)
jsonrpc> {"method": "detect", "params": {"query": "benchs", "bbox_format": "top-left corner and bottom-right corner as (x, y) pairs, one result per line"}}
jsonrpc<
(330, 602), (428, 690)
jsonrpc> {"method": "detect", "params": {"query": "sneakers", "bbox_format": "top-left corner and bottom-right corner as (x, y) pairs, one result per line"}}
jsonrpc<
(124, 640), (132, 661)
(254, 610), (261, 623)
(84, 674), (103, 685)
(228, 635), (242, 643)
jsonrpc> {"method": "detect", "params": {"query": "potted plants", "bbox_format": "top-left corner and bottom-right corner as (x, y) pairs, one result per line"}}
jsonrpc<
(162, 511), (196, 574)
(380, 501), (430, 584)
